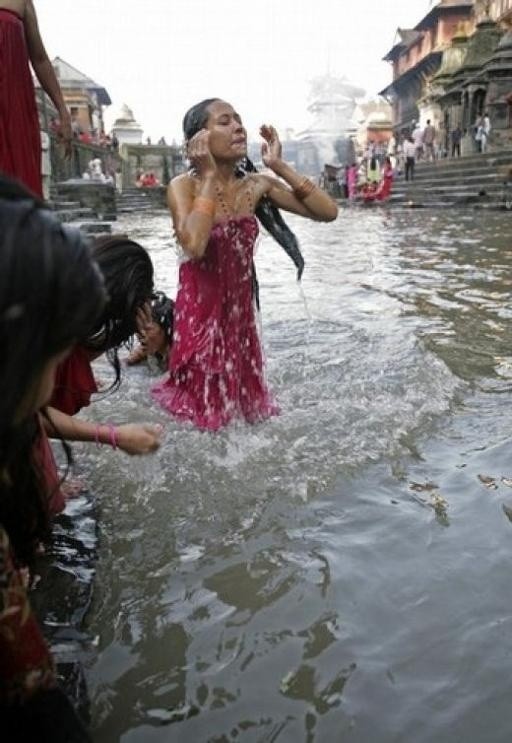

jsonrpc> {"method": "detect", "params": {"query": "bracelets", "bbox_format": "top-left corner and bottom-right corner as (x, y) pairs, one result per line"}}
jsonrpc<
(93, 424), (103, 451)
(107, 425), (119, 449)
(293, 177), (316, 198)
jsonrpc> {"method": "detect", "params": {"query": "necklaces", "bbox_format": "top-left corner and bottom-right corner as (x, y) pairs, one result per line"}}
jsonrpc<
(212, 177), (256, 249)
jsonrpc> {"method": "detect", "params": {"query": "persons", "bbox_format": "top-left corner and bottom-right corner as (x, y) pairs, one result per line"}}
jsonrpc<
(47, 116), (187, 196)
(1, 0), (75, 202)
(41, 233), (164, 455)
(126, 292), (174, 372)
(2, 174), (109, 741)
(339, 112), (492, 205)
(151, 97), (338, 433)
